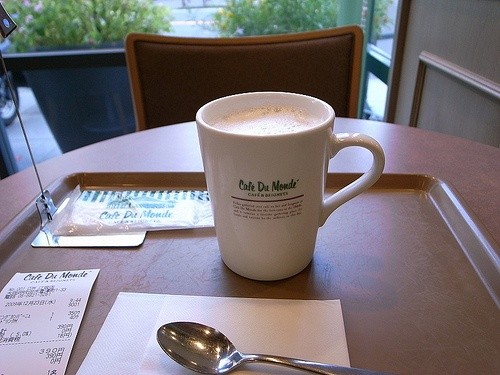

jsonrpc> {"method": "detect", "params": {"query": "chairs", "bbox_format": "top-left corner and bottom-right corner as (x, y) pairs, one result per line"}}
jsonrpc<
(124, 25), (363, 131)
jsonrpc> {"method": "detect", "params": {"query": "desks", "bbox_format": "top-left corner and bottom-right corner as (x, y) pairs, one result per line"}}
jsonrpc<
(0, 118), (500, 373)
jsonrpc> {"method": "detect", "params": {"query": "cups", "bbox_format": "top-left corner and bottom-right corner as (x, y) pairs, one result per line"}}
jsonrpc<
(196, 91), (385, 281)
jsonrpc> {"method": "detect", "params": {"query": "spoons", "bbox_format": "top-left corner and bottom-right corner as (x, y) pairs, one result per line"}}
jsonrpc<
(157, 320), (394, 375)
